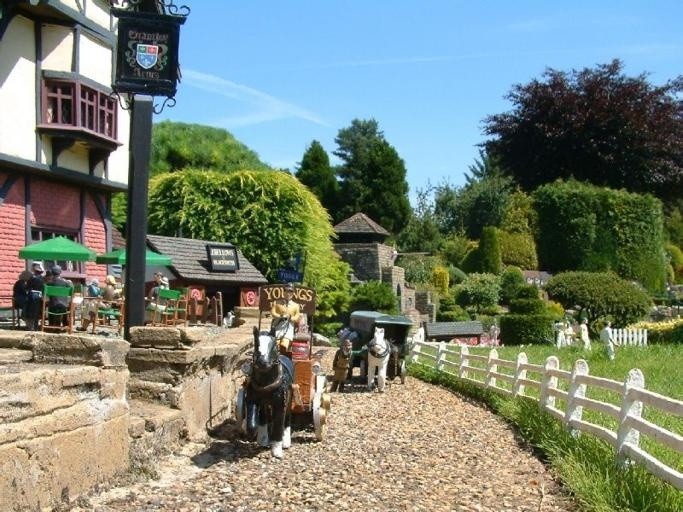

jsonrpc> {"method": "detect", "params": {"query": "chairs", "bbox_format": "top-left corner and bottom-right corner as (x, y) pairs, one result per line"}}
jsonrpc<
(11, 281), (190, 336)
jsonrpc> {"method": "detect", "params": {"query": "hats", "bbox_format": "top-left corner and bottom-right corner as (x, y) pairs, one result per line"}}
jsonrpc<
(19, 264), (62, 280)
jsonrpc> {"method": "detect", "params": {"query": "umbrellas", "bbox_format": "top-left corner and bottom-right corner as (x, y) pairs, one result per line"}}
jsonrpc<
(19, 235), (96, 262)
(96, 246), (172, 267)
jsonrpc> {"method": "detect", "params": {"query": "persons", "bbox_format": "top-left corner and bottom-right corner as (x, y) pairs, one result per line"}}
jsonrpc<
(11, 262), (123, 333)
(329, 339), (353, 394)
(144, 272), (170, 326)
(221, 306), (241, 328)
(269, 282), (299, 355)
(554, 316), (593, 352)
(600, 320), (622, 363)
(649, 281), (678, 324)
